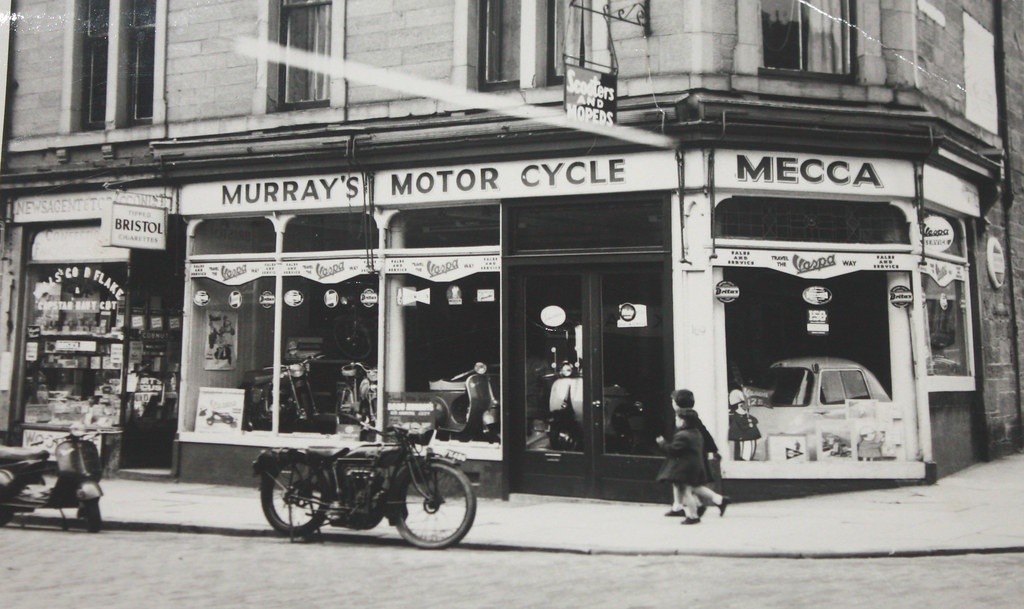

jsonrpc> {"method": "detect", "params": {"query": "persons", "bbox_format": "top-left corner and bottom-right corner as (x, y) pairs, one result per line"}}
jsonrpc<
(219, 321), (235, 365)
(655, 408), (729, 524)
(665, 389), (718, 518)
(38, 293), (59, 314)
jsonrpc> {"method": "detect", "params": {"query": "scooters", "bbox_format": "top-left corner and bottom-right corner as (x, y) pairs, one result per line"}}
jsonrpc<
(428, 361), (500, 444)
(549, 347), (630, 451)
(0, 422), (123, 534)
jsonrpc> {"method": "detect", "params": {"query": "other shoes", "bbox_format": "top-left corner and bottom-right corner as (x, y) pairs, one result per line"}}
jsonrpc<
(681, 516), (701, 524)
(664, 508), (685, 518)
(717, 496), (729, 516)
(697, 505), (708, 517)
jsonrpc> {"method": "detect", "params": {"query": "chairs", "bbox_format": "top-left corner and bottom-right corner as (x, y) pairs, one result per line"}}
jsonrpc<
(453, 372), (499, 444)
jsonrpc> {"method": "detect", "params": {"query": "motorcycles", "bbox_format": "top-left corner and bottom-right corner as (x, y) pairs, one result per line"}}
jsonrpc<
(252, 405), (477, 550)
(238, 352), (327, 432)
(336, 361), (377, 428)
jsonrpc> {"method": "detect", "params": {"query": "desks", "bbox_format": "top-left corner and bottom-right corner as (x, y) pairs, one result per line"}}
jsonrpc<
(401, 391), (468, 432)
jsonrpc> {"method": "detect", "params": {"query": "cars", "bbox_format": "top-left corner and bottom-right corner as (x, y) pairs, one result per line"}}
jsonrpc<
(749, 356), (892, 461)
